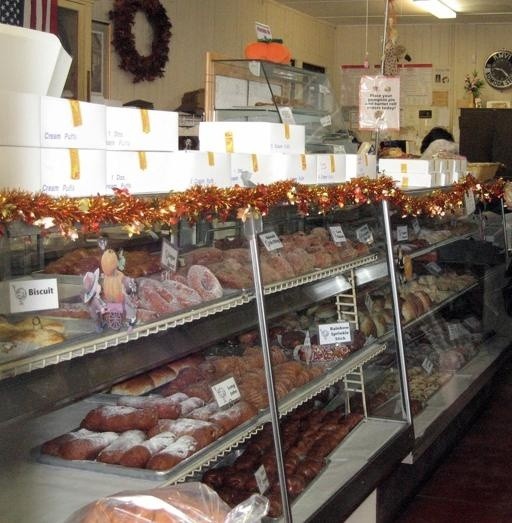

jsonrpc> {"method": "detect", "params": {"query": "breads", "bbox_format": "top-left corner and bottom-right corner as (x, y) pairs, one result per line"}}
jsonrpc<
(0, 214), (485, 522)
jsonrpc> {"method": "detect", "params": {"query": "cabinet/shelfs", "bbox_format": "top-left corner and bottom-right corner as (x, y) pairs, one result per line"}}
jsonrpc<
(458, 108), (512, 182)
(203, 50), (327, 155)
(0, 179), (512, 523)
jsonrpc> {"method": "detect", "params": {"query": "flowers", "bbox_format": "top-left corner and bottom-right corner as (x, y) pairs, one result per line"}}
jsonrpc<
(462, 69), (484, 97)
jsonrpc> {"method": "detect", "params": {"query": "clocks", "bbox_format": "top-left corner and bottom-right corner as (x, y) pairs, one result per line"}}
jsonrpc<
(483, 49), (512, 89)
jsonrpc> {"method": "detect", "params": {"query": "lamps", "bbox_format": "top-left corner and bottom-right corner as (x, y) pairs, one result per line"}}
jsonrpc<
(412, 0), (458, 20)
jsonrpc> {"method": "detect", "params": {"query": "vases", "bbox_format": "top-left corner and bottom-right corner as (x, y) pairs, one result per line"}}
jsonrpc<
(472, 93), (478, 109)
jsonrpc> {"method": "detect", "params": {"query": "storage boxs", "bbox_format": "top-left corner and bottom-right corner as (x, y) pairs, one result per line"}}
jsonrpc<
(2, 89), (318, 198)
(1, 24), (74, 98)
(317, 154), (468, 192)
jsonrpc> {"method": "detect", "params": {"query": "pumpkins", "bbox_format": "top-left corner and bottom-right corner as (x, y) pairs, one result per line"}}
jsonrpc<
(245, 37), (293, 64)
